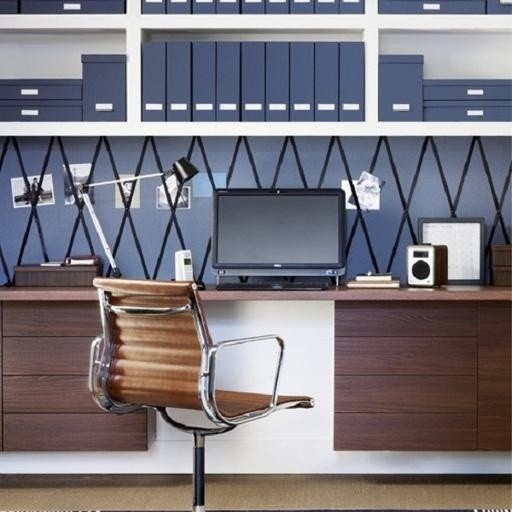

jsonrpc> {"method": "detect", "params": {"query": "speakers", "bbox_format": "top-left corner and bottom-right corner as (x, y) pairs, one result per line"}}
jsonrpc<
(406, 244), (449, 288)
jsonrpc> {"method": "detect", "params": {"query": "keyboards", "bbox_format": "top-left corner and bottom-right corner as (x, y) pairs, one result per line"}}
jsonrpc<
(215, 284), (328, 292)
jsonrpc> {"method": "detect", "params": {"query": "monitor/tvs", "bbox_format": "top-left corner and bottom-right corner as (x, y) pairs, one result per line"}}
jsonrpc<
(212, 188), (347, 286)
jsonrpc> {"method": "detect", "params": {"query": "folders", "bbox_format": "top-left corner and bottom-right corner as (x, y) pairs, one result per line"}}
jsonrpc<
(141, 41), (365, 122)
(142, 0), (364, 14)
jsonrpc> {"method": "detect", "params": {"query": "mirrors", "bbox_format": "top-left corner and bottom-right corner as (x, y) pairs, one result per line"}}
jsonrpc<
(417, 217), (486, 286)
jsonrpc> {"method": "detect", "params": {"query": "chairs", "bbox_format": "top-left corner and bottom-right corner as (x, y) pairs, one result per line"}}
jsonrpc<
(88, 278), (314, 512)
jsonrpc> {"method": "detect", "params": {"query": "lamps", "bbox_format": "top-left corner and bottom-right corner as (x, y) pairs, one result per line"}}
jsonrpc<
(80, 157), (199, 278)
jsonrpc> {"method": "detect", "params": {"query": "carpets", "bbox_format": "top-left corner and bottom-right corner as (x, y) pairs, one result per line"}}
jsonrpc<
(300, 509), (512, 512)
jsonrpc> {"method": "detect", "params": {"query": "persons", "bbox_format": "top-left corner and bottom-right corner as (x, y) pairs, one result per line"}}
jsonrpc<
(32, 178), (45, 203)
(23, 182), (31, 205)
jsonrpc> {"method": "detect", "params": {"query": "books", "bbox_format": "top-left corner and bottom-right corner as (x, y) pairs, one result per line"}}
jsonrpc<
(355, 272), (392, 281)
(346, 277), (401, 289)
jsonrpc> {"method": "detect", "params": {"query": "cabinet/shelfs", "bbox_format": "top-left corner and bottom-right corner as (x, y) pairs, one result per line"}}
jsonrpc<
(0, 0), (512, 137)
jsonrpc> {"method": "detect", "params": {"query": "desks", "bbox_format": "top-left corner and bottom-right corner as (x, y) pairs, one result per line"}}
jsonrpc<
(0, 283), (512, 476)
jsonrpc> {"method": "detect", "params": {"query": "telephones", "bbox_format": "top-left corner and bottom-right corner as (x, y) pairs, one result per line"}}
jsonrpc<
(175, 250), (193, 282)
